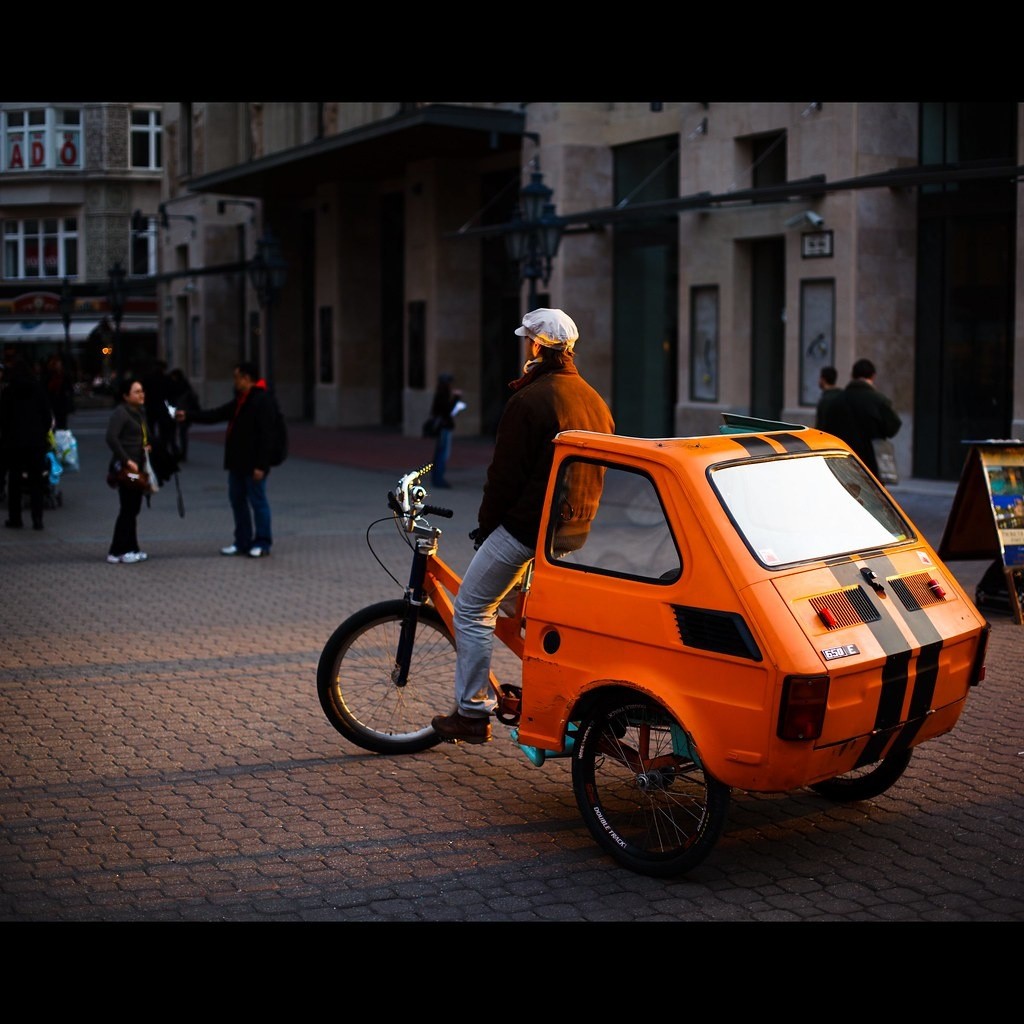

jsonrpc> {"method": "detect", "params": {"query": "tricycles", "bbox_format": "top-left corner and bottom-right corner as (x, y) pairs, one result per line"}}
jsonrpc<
(317, 414), (999, 870)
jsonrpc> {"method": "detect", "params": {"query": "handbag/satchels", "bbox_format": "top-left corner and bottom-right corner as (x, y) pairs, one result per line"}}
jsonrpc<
(55, 429), (80, 474)
(421, 419), (444, 440)
(142, 445), (180, 497)
(872, 436), (899, 486)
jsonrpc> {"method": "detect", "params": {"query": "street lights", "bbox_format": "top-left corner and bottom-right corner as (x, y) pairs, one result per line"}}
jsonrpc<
(105, 262), (129, 407)
(58, 280), (78, 353)
(505, 170), (563, 311)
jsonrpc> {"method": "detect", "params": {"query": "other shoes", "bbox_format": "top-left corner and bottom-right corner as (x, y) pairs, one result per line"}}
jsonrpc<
(32, 521), (44, 530)
(249, 546), (263, 556)
(220, 544), (238, 554)
(108, 552), (147, 563)
(4, 519), (24, 529)
(431, 479), (454, 491)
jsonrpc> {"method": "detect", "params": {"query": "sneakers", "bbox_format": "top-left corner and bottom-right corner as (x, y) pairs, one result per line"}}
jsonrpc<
(432, 711), (493, 744)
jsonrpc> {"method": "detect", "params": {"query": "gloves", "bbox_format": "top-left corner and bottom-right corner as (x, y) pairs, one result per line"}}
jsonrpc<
(469, 523), (495, 550)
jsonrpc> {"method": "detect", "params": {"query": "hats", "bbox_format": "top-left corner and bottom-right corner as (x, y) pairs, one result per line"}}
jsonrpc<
(514, 307), (579, 351)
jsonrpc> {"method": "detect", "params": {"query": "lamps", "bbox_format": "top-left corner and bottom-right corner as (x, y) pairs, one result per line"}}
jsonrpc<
(215, 198), (257, 216)
(132, 209), (198, 231)
(471, 127), (541, 158)
(782, 207), (825, 230)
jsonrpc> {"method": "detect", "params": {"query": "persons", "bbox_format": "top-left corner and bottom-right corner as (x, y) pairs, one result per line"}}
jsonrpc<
(428, 379), (463, 489)
(175, 362), (273, 559)
(0, 357), (200, 530)
(814, 357), (903, 488)
(430, 308), (615, 745)
(104, 380), (156, 565)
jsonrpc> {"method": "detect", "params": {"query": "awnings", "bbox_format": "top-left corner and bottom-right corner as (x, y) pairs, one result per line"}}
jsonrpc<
(449, 158), (1024, 245)
